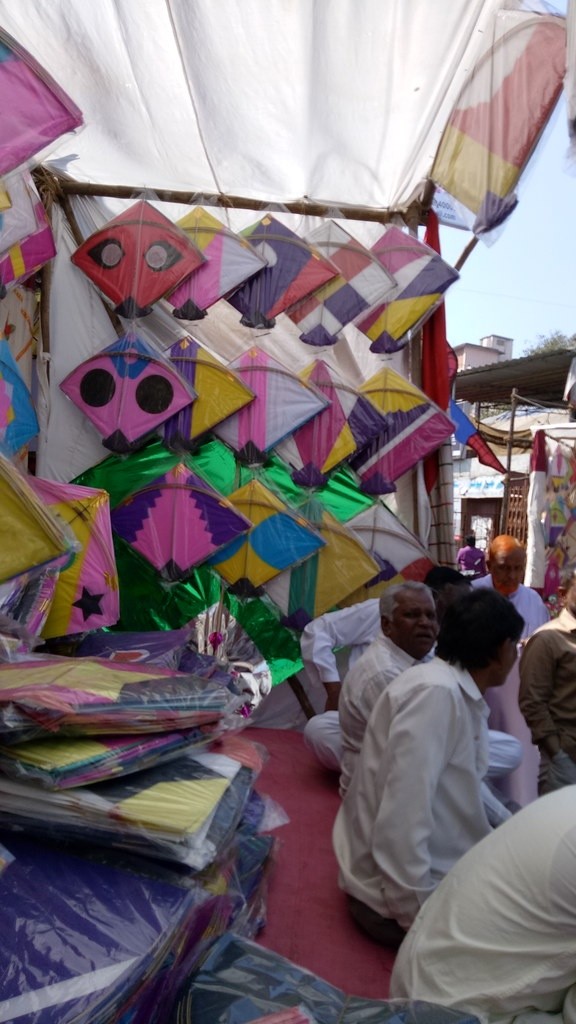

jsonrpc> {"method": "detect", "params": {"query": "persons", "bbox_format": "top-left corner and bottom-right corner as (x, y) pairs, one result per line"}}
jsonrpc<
(390, 779), (576, 1024)
(519, 577), (575, 798)
(455, 534), (487, 581)
(467, 535), (550, 816)
(338, 578), (513, 830)
(299, 565), (524, 781)
(331, 587), (527, 951)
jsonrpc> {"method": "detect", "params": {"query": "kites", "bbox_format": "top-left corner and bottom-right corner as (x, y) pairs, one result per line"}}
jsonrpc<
(0, 28), (576, 1023)
